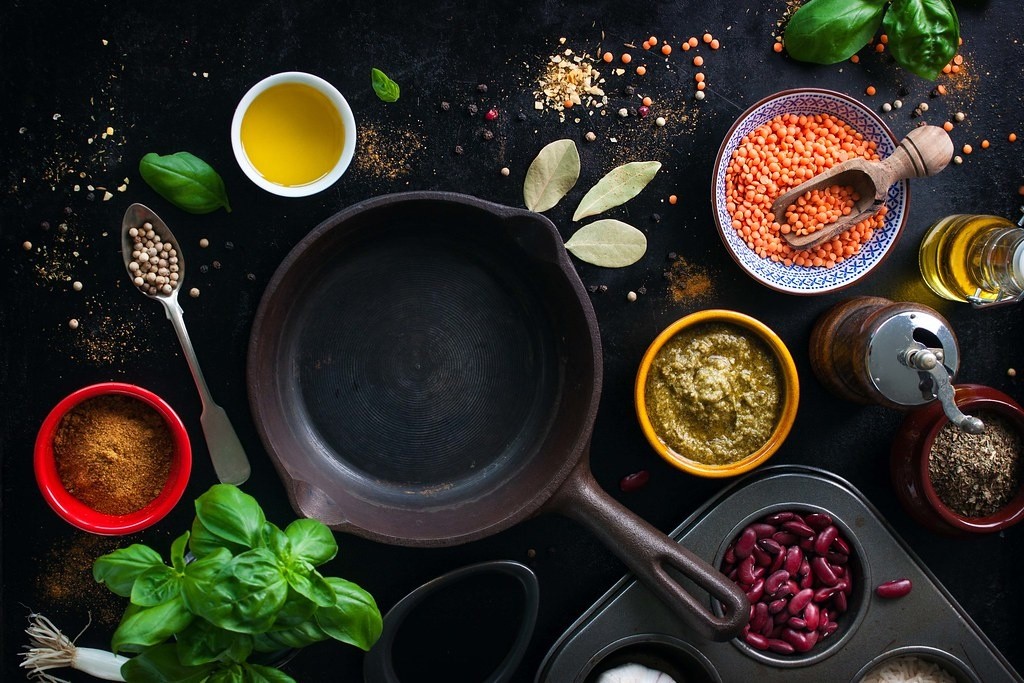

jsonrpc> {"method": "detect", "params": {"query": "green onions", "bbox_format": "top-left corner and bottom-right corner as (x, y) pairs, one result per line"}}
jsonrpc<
(14, 612), (132, 683)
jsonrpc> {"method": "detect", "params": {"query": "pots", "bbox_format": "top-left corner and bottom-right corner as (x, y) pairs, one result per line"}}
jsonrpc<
(250, 193), (751, 643)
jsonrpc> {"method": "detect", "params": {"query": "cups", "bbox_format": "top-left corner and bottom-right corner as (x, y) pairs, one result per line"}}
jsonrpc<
(893, 383), (1024, 539)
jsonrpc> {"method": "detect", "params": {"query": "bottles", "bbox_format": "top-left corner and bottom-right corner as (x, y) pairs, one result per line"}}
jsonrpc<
(807, 296), (984, 438)
(918, 212), (1024, 309)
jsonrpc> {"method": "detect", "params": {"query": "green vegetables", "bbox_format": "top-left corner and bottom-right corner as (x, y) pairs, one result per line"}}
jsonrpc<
(92, 484), (383, 683)
(371, 68), (400, 103)
(782, 0), (961, 83)
(140, 151), (232, 214)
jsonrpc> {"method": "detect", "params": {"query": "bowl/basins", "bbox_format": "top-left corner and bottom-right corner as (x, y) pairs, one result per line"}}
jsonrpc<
(711, 87), (911, 298)
(231, 71), (355, 197)
(33, 382), (191, 537)
(634, 309), (799, 476)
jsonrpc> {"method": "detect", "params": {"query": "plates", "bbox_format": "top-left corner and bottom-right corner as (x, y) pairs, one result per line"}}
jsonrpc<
(361, 561), (540, 683)
(535, 464), (1024, 683)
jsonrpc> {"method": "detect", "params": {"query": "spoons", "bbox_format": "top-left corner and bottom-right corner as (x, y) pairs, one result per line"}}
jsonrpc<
(123, 202), (251, 491)
